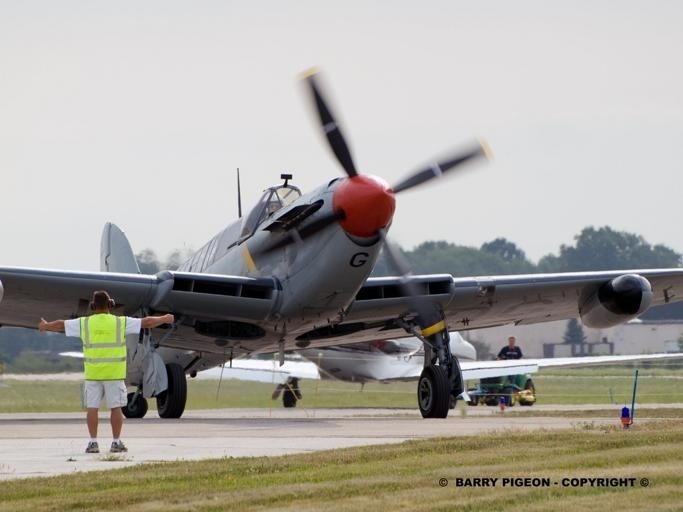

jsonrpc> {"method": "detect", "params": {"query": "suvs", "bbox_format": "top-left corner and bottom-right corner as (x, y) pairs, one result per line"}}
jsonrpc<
(467, 364), (538, 408)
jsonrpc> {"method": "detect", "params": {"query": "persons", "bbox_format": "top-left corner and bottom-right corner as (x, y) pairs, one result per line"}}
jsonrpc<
(496, 337), (524, 406)
(261, 202), (279, 220)
(37, 290), (174, 453)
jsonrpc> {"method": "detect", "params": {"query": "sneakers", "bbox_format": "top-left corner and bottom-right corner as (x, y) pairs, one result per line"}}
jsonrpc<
(110, 440), (127, 453)
(86, 442), (99, 453)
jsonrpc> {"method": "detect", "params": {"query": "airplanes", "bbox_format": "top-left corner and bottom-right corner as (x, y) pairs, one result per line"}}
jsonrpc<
(0, 66), (683, 417)
(58, 330), (681, 417)
